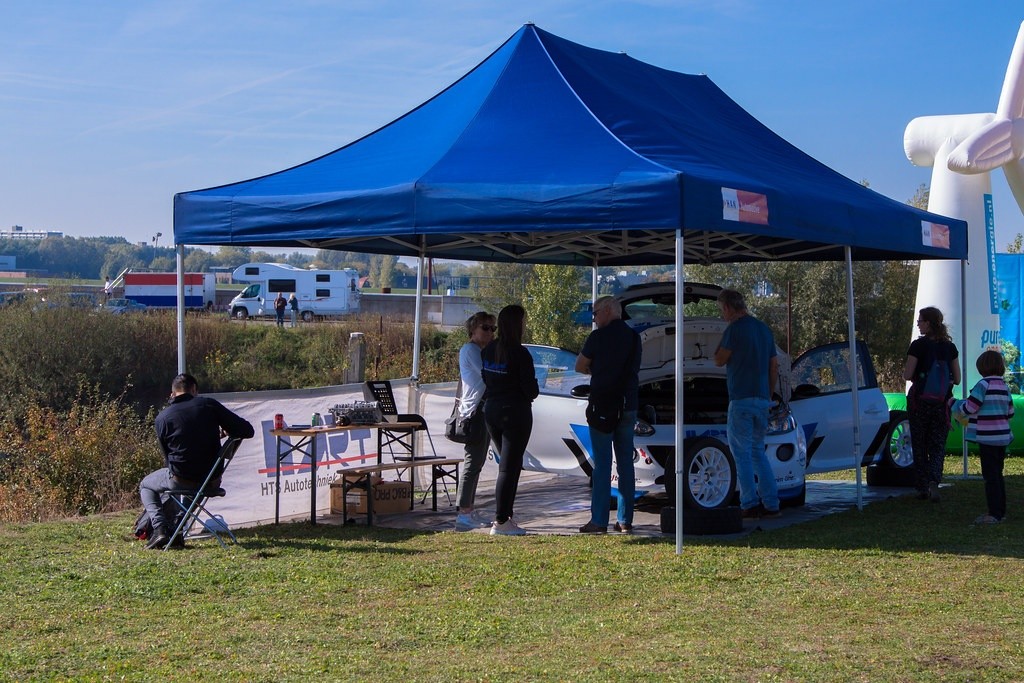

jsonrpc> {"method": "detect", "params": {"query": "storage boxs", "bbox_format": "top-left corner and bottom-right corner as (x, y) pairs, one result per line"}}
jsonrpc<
(330, 475), (411, 515)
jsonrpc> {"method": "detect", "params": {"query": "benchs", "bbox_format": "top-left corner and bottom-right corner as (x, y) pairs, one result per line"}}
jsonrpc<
(339, 458), (465, 525)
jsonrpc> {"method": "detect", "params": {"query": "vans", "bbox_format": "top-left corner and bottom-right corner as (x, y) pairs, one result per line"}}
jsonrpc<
(226, 262), (361, 321)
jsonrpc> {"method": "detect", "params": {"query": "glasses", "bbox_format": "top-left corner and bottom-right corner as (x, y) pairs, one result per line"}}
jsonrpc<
(476, 324), (497, 332)
(593, 307), (606, 316)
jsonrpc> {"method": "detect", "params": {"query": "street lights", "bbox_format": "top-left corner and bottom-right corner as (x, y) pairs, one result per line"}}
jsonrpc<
(152, 232), (162, 259)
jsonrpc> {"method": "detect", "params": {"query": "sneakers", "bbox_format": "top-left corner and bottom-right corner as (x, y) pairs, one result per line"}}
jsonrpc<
(920, 489), (929, 498)
(766, 508), (782, 518)
(742, 505), (764, 519)
(470, 508), (493, 528)
(489, 516), (526, 536)
(454, 512), (487, 532)
(167, 532), (184, 549)
(580, 520), (607, 534)
(613, 521), (633, 532)
(143, 528), (167, 550)
(929, 481), (941, 503)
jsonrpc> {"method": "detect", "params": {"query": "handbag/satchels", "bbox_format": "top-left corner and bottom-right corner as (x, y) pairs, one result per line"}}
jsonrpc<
(586, 390), (623, 433)
(444, 406), (478, 444)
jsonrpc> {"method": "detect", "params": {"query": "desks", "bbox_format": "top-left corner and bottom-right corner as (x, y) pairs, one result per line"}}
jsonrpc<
(271, 421), (423, 527)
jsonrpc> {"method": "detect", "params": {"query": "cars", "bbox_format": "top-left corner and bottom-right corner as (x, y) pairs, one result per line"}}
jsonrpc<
(882, 371), (1023, 489)
(102, 298), (148, 316)
(548, 301), (593, 326)
(44, 292), (98, 313)
(490, 280), (889, 510)
(0, 292), (27, 307)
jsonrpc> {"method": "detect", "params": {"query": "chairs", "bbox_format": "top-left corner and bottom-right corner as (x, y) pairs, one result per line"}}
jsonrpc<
(164, 436), (242, 551)
(386, 414), (458, 510)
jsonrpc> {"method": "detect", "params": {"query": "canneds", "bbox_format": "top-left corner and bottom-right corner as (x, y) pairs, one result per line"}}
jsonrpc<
(274, 413), (284, 429)
(312, 412), (320, 426)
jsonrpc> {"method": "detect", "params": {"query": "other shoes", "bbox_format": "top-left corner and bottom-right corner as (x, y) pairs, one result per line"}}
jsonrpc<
(982, 513), (1000, 524)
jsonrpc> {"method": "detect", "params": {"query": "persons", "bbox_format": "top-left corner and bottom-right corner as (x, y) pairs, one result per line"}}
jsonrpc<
(104, 275), (114, 300)
(140, 373), (254, 552)
(574, 296), (642, 532)
(452, 310), (496, 530)
(273, 292), (287, 330)
(712, 288), (780, 519)
(479, 303), (540, 535)
(287, 292), (299, 327)
(902, 307), (961, 502)
(958, 350), (1015, 526)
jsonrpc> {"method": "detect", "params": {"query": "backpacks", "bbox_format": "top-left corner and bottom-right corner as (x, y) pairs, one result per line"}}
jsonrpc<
(922, 358), (951, 411)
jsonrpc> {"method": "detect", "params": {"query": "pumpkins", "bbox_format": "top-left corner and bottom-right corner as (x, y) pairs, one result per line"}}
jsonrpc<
(335, 411), (351, 426)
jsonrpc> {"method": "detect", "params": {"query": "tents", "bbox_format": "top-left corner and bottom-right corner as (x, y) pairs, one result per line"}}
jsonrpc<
(169, 16), (956, 538)
